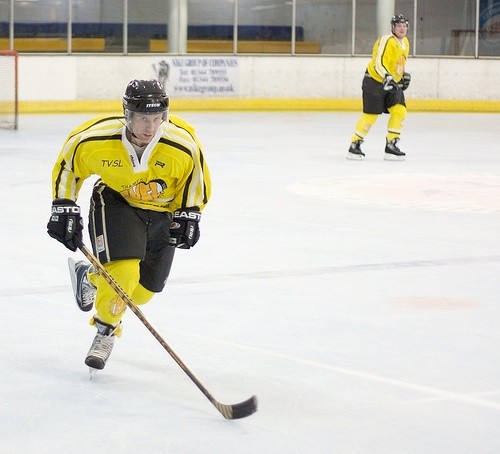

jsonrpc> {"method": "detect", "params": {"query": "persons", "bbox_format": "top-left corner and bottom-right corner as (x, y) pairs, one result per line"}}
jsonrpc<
(48, 79), (213, 368)
(348, 14), (411, 156)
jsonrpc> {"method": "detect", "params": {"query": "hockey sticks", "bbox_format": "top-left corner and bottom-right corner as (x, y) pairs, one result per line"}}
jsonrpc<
(382, 73), (404, 91)
(72, 229), (259, 421)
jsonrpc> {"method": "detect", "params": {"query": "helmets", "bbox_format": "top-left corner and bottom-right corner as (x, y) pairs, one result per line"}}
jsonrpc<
(122, 79), (169, 112)
(391, 13), (408, 23)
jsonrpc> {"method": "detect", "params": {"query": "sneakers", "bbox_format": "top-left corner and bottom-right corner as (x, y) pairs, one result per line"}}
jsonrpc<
(85, 318), (122, 382)
(384, 137), (406, 161)
(346, 140), (365, 159)
(67, 256), (97, 311)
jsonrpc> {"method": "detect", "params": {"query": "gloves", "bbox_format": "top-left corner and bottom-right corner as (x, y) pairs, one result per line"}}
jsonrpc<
(382, 73), (399, 94)
(168, 206), (201, 249)
(47, 199), (83, 252)
(401, 73), (411, 90)
